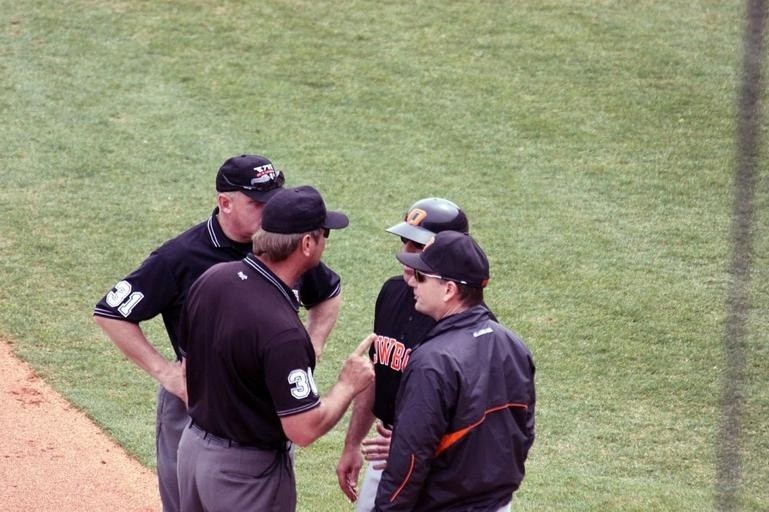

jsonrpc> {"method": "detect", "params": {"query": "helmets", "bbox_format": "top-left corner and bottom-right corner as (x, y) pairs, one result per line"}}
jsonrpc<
(385, 196), (467, 248)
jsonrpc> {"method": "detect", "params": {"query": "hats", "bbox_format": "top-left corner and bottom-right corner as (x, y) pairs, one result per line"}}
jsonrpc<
(394, 230), (490, 283)
(216, 155), (286, 203)
(261, 186), (349, 234)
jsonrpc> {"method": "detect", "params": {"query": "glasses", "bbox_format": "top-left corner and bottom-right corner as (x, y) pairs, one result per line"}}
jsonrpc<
(400, 235), (425, 250)
(321, 228), (331, 238)
(413, 268), (468, 286)
(242, 169), (285, 193)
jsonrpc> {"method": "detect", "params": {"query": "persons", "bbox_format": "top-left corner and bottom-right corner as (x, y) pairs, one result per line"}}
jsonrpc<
(90, 152), (341, 511)
(370, 231), (536, 512)
(336, 197), (469, 511)
(175, 186), (378, 511)
